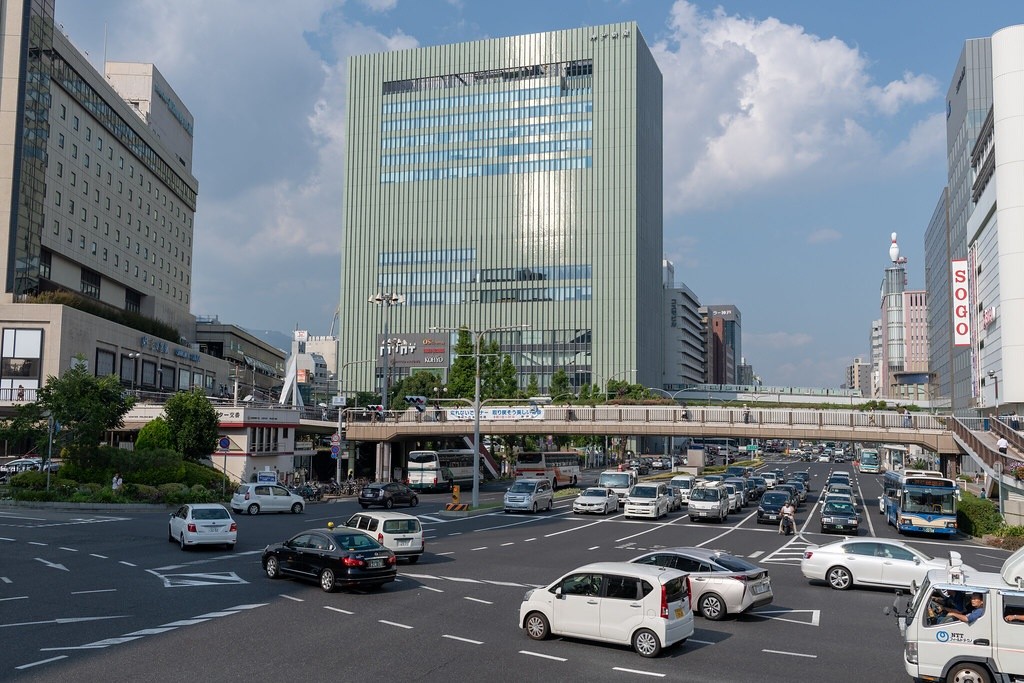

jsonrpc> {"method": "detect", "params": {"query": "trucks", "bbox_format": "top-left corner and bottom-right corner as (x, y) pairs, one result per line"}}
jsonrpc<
(594, 468), (638, 509)
(882, 544), (1024, 683)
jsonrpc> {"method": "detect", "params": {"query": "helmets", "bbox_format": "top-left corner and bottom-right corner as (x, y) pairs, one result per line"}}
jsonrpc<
(785, 501), (790, 504)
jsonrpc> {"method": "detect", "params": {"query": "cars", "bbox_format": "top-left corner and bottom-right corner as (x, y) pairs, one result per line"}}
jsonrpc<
(800, 537), (977, 591)
(763, 439), (854, 464)
(755, 490), (792, 525)
(693, 466), (857, 520)
(0, 459), (59, 475)
(358, 481), (418, 509)
(628, 456), (681, 475)
(667, 486), (683, 514)
(169, 502), (238, 551)
(231, 483), (306, 516)
(622, 547), (774, 622)
(820, 499), (860, 534)
(261, 521), (398, 593)
(877, 492), (884, 515)
(572, 487), (618, 516)
(519, 560), (695, 659)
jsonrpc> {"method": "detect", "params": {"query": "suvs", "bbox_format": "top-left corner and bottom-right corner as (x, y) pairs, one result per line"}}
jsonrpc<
(337, 511), (424, 564)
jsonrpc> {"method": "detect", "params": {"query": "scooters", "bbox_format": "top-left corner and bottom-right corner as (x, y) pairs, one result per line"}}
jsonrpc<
(777, 508), (797, 536)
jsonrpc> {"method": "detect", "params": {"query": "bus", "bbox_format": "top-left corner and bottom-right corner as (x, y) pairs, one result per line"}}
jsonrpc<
(516, 451), (581, 491)
(883, 468), (962, 538)
(407, 449), (484, 493)
(860, 447), (881, 474)
(690, 437), (742, 458)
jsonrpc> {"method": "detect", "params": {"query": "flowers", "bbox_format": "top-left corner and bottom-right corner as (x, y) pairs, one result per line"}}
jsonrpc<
(1005, 463), (1024, 482)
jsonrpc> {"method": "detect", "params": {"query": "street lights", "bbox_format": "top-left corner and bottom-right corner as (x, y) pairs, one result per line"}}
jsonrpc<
(432, 386), (449, 408)
(428, 325), (534, 508)
(709, 396), (742, 466)
(336, 358), (378, 485)
(903, 385), (934, 416)
(367, 292), (406, 417)
(128, 352), (141, 392)
(986, 369), (999, 420)
(576, 369), (639, 470)
(645, 387), (698, 473)
(746, 395), (769, 458)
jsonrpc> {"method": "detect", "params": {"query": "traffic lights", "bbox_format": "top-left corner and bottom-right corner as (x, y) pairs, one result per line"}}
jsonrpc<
(367, 404), (383, 413)
(415, 404), (426, 412)
(403, 395), (427, 405)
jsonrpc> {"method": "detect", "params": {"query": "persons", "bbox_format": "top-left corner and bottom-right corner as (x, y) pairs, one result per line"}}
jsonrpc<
(566, 402), (573, 420)
(997, 435), (1009, 465)
(1005, 615), (1024, 621)
(322, 407), (328, 420)
(975, 471), (979, 485)
(903, 406), (909, 427)
(779, 501), (797, 536)
(681, 403), (689, 420)
(112, 473), (122, 494)
(980, 488), (986, 498)
(743, 405), (751, 422)
(433, 404), (441, 421)
(210, 392), (230, 404)
(825, 504), (834, 512)
(363, 406), (380, 421)
(933, 590), (983, 623)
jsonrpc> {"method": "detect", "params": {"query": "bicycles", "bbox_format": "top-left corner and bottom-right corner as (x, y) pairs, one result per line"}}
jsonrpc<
(286, 477), (370, 502)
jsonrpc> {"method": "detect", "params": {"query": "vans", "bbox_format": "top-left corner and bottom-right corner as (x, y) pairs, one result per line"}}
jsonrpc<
(687, 480), (730, 524)
(623, 482), (670, 521)
(504, 479), (554, 514)
(669, 475), (695, 504)
